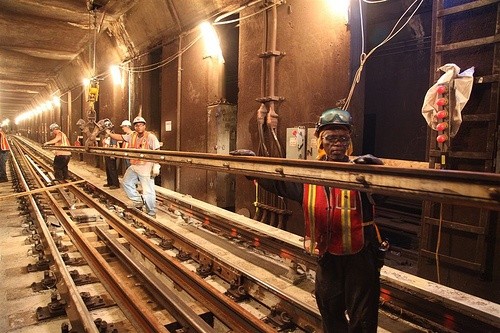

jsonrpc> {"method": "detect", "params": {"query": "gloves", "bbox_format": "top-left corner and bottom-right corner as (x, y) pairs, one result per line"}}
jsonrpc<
(152, 163), (160, 176)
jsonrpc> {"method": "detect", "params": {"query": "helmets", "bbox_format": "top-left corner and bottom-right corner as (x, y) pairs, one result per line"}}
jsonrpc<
(120, 120), (131, 127)
(316, 108), (353, 138)
(133, 116), (146, 125)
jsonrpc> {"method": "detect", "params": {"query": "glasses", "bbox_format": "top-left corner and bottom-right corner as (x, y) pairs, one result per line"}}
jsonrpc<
(321, 109), (350, 123)
(322, 134), (349, 146)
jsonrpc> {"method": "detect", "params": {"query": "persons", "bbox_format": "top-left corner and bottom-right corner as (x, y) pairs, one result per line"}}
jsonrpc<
(0, 128), (10, 183)
(105, 116), (161, 217)
(43, 123), (72, 180)
(101, 121), (120, 189)
(230, 109), (384, 333)
(119, 119), (138, 189)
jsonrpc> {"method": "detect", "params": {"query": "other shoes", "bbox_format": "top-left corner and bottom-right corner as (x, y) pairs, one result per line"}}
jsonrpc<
(149, 215), (156, 220)
(53, 177), (63, 181)
(104, 183), (113, 186)
(109, 185), (119, 189)
(0, 178), (8, 183)
(137, 206), (143, 212)
